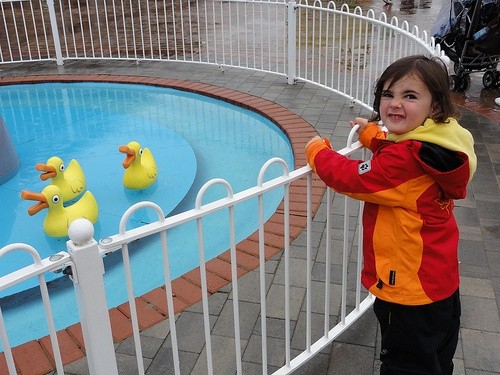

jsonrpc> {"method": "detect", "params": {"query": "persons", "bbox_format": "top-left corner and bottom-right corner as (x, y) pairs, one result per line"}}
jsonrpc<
(302, 53), (479, 374)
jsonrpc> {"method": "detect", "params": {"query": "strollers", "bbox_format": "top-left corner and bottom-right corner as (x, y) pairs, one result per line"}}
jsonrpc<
(430, 0), (500, 91)
